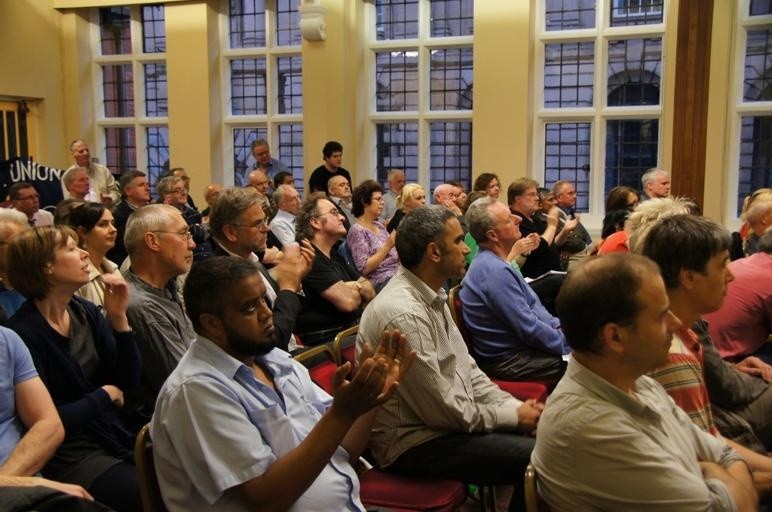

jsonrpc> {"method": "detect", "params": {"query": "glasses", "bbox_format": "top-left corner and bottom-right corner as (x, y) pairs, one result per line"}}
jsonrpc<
(314, 208), (338, 218)
(231, 215), (269, 227)
(369, 196), (380, 200)
(20, 194), (40, 200)
(154, 228), (192, 240)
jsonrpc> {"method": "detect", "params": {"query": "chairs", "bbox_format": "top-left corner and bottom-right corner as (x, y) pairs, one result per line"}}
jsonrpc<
(284, 345), (497, 512)
(333, 322), (549, 511)
(133, 414), (415, 512)
(448, 283), (555, 386)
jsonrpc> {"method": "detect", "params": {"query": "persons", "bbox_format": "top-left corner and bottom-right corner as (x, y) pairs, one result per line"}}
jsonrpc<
(326, 175), (357, 237)
(701, 228), (772, 358)
(639, 167), (670, 204)
(464, 191), (541, 276)
(506, 177), (580, 278)
(183, 186), (315, 351)
(459, 195), (572, 380)
(273, 171), (296, 188)
(105, 169), (151, 268)
(167, 167), (196, 210)
(268, 183), (302, 248)
(200, 184), (223, 222)
(0, 322), (111, 512)
(385, 184), (426, 233)
(104, 204), (196, 395)
(157, 176), (213, 257)
(3, 225), (147, 511)
(250, 139), (288, 182)
(307, 141), (352, 194)
(152, 256), (417, 512)
(474, 172), (501, 199)
(533, 187), (586, 252)
(433, 181), (467, 229)
(248, 170), (275, 217)
(60, 138), (121, 209)
(603, 186), (640, 215)
(596, 211), (629, 255)
(294, 192), (376, 338)
(553, 180), (592, 244)
(10, 182), (53, 226)
(345, 179), (396, 292)
(627, 195), (772, 453)
(378, 169), (406, 226)
(63, 168), (90, 200)
(69, 202), (123, 317)
(353, 206), (544, 484)
(739, 187), (772, 255)
(531, 252), (772, 512)
(0, 206), (30, 321)
(642, 214), (772, 489)
